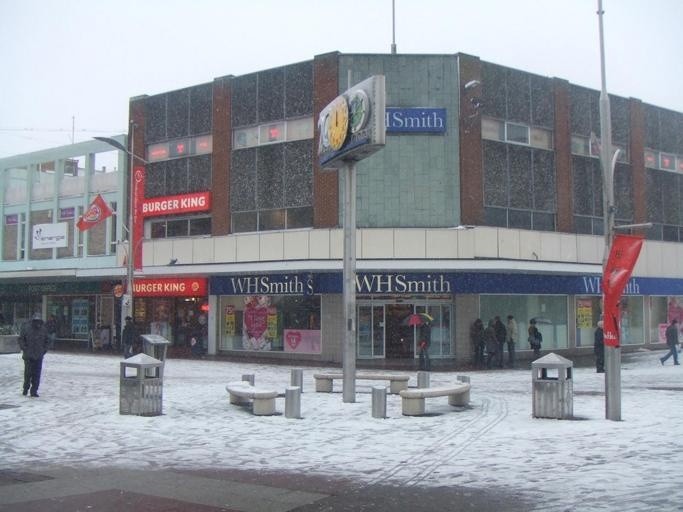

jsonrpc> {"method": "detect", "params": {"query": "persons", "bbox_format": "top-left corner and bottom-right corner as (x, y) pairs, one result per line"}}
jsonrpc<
(505, 315), (518, 368)
(43, 315), (57, 350)
(483, 319), (503, 369)
(527, 319), (541, 361)
(592, 320), (604, 373)
(493, 316), (506, 367)
(416, 322), (432, 371)
(17, 312), (51, 397)
(122, 316), (135, 358)
(469, 318), (485, 368)
(659, 319), (681, 366)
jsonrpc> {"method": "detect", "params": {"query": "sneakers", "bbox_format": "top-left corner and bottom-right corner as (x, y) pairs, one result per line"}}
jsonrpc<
(661, 358), (664, 365)
(674, 362), (680, 365)
(31, 394), (39, 397)
(24, 389), (28, 395)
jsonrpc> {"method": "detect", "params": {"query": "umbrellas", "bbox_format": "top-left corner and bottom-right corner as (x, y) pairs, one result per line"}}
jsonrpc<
(399, 312), (433, 329)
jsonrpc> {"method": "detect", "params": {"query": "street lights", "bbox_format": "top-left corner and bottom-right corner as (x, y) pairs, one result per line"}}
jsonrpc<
(92, 119), (151, 326)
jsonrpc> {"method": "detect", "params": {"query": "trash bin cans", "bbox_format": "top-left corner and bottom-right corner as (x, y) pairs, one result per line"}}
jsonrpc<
(120, 353), (164, 416)
(138, 334), (169, 378)
(531, 352), (573, 420)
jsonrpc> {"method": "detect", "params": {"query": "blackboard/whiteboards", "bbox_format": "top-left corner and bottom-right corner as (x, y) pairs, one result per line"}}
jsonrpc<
(90, 329), (103, 347)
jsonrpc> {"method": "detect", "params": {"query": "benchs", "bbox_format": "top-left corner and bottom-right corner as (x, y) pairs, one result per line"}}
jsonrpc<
(399, 381), (471, 417)
(312, 371), (410, 395)
(225, 382), (279, 416)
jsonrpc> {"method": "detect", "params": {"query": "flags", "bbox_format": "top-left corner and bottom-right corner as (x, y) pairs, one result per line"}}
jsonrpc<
(602, 233), (647, 347)
(76, 195), (112, 230)
